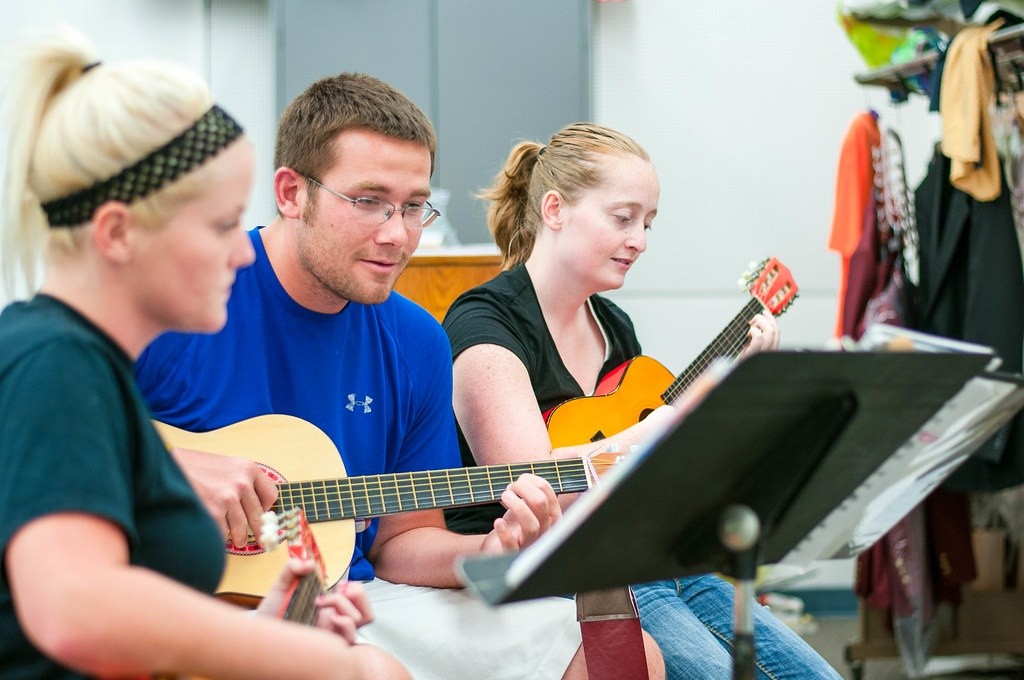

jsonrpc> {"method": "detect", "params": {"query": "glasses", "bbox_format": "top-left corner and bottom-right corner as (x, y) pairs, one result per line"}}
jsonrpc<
(304, 176), (441, 230)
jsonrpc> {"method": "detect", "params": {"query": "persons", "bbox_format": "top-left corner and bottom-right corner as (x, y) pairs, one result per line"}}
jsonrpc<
(0, 46), (412, 680)
(441, 123), (850, 680)
(128, 72), (665, 680)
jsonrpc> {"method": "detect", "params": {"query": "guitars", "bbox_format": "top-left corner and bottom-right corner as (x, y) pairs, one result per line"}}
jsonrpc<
(271, 507), (329, 629)
(149, 408), (623, 607)
(542, 256), (799, 453)
